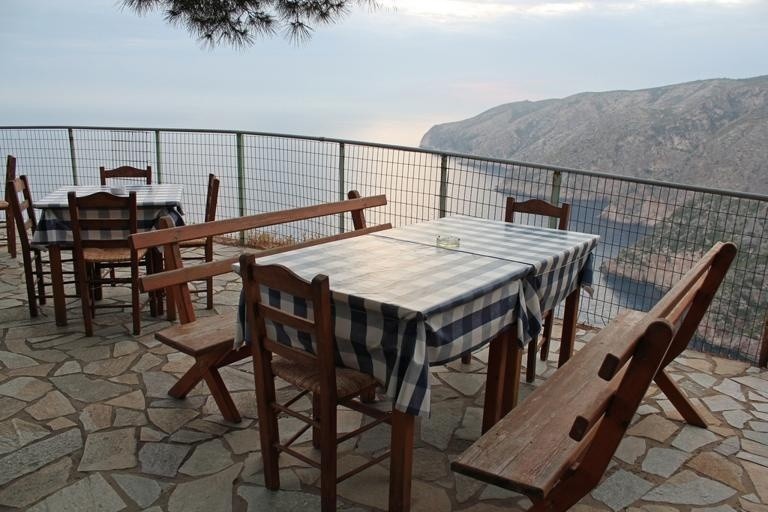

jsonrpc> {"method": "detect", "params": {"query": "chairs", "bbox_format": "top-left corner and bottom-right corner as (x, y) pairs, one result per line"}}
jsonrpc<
(0, 154), (220, 336)
(237, 253), (392, 511)
(461, 196), (571, 368)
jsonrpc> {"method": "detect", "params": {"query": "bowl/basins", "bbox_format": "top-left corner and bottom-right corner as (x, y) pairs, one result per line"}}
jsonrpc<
(110, 185), (125, 195)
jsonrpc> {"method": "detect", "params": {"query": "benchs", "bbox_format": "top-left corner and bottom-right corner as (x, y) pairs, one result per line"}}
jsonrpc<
(126, 189), (392, 423)
(451, 240), (738, 512)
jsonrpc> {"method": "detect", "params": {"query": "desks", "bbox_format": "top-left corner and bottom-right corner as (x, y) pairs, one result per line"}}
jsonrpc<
(231, 232), (533, 512)
(369, 212), (601, 421)
